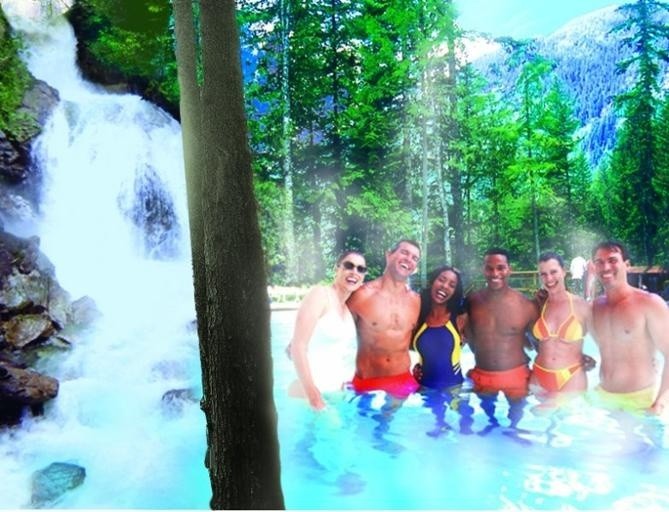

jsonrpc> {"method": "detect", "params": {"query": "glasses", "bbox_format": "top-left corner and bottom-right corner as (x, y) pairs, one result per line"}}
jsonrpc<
(341, 261), (369, 274)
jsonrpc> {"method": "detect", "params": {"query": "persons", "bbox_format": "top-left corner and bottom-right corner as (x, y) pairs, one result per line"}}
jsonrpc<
(523, 248), (591, 393)
(568, 253), (589, 297)
(411, 247), (597, 447)
(410, 264), (475, 441)
(288, 249), (369, 413)
(533, 238), (669, 418)
(344, 237), (423, 459)
